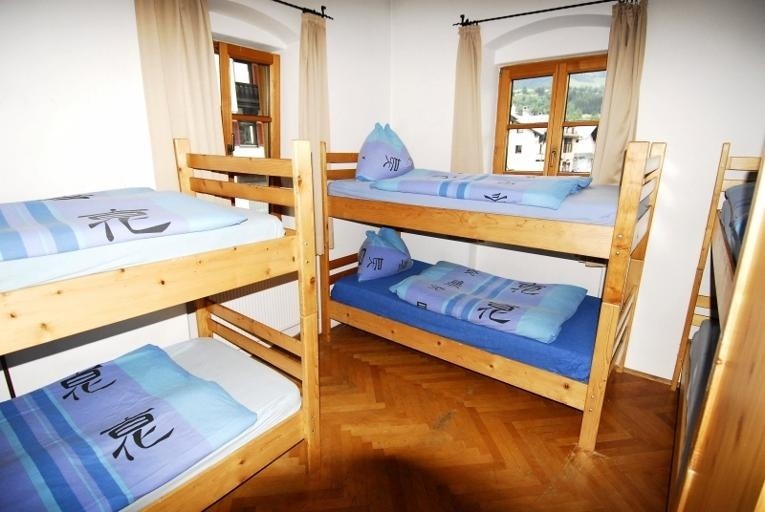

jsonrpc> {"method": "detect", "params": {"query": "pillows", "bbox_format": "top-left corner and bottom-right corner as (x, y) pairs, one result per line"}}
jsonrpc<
(356, 225), (413, 280)
(354, 124), (415, 181)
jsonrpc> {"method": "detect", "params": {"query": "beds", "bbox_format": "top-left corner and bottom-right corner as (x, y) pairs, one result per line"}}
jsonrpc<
(319, 137), (667, 453)
(667, 138), (765, 511)
(0, 138), (318, 512)
